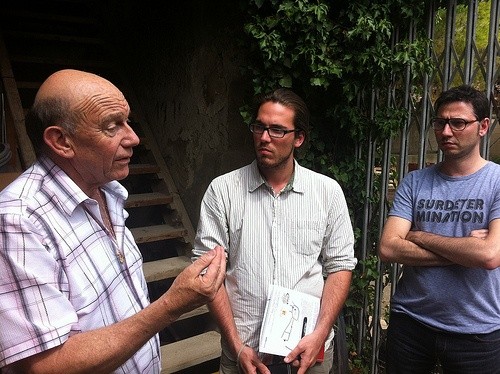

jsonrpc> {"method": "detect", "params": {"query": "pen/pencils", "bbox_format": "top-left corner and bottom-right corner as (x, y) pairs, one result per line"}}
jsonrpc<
(298, 317), (307, 360)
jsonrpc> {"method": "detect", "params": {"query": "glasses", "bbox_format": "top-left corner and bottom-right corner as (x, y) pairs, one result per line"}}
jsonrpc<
(430, 118), (480, 131)
(249, 122), (302, 138)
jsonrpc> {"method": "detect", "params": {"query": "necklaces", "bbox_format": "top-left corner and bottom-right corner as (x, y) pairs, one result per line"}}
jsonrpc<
(89, 189), (125, 264)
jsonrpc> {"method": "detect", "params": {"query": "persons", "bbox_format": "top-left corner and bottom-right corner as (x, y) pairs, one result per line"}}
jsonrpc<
(377, 84), (500, 374)
(0, 68), (227, 374)
(189, 88), (357, 374)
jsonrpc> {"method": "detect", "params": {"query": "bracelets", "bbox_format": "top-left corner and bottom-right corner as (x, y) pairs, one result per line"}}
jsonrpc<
(235, 344), (248, 370)
